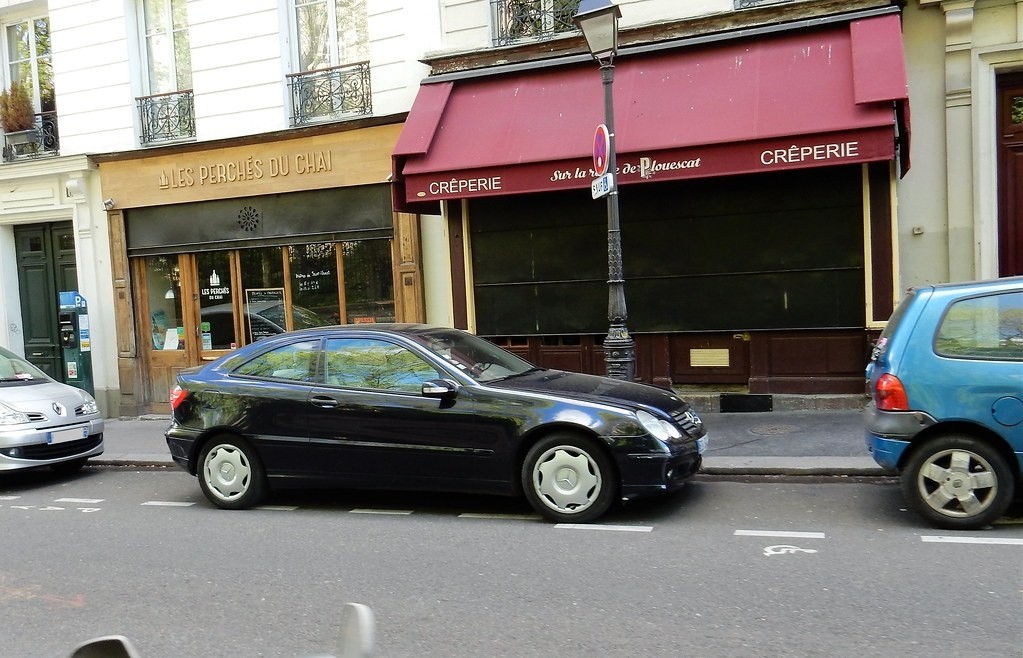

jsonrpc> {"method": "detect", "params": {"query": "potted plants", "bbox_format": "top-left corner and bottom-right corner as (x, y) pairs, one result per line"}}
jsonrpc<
(0, 80), (39, 146)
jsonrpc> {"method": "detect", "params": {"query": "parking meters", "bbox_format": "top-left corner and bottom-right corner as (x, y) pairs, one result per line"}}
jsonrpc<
(59, 291), (94, 399)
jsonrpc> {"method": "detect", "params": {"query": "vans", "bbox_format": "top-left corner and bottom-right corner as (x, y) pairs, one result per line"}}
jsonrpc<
(864, 276), (1023, 530)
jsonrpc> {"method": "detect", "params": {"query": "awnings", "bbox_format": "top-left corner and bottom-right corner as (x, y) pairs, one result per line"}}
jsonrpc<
(392, 14), (912, 216)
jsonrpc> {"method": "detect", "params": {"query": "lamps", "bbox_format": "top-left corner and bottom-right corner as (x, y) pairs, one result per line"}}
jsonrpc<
(104, 199), (114, 210)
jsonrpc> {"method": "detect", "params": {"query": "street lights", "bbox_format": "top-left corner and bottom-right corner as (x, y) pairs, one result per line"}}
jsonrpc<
(571, 0), (623, 384)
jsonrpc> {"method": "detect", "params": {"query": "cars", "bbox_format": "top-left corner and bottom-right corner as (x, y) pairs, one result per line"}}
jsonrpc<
(164, 323), (709, 523)
(201, 300), (333, 349)
(0, 346), (104, 474)
